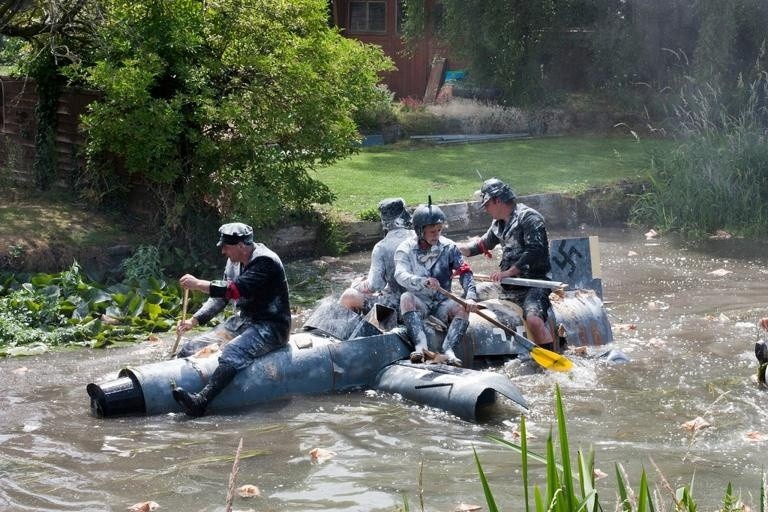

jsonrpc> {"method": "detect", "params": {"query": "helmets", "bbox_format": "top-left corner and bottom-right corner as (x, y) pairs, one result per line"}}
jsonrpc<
(412, 204), (445, 240)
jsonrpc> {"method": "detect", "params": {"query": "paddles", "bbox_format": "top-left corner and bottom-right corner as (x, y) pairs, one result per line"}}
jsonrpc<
(424, 281), (573, 371)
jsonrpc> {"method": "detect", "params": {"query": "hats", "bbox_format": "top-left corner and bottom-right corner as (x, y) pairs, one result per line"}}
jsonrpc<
(477, 177), (516, 210)
(377, 197), (412, 231)
(215, 222), (254, 247)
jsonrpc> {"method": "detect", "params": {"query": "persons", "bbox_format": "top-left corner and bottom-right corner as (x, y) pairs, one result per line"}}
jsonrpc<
(453, 178), (553, 357)
(393, 204), (480, 368)
(334, 197), (413, 324)
(172, 219), (292, 418)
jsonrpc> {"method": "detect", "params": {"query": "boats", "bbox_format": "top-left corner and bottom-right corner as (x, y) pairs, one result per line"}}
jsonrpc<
(86, 236), (614, 426)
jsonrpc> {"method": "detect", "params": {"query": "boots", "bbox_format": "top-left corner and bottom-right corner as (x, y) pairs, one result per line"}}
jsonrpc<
(171, 357), (239, 419)
(439, 317), (469, 367)
(402, 310), (429, 363)
